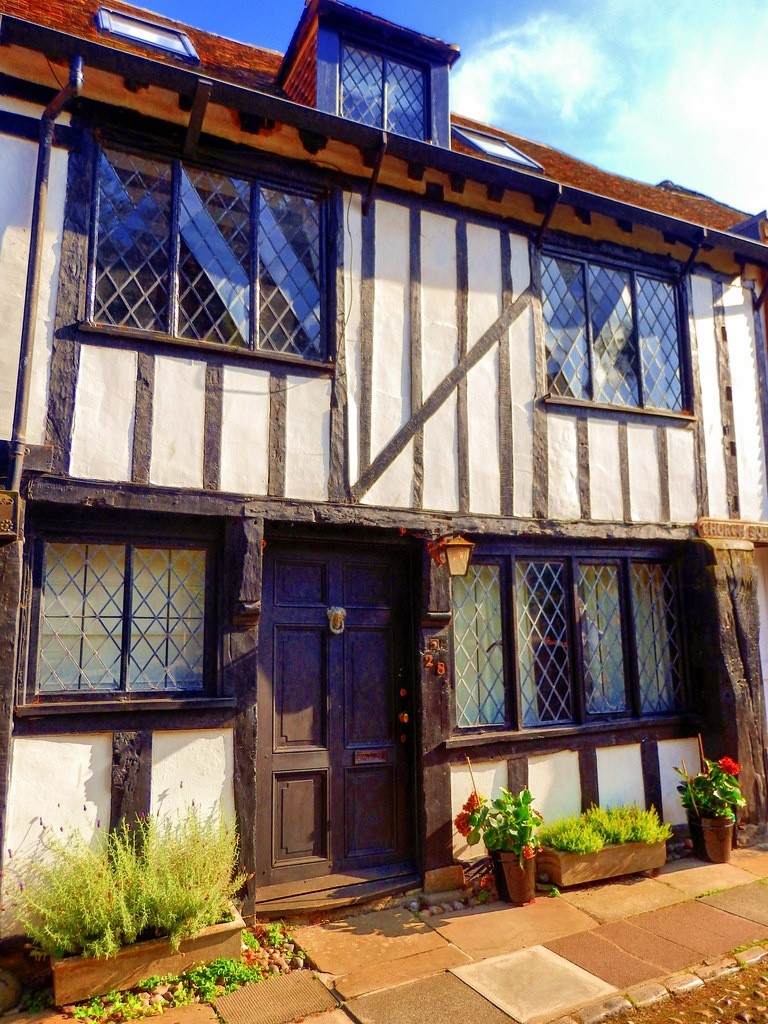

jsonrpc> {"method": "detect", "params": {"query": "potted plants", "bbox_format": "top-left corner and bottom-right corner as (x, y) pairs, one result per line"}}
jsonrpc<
(0, 779), (256, 1006)
(534, 799), (674, 887)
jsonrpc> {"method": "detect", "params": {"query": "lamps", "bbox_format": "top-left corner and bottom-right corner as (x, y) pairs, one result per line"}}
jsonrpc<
(419, 530), (476, 578)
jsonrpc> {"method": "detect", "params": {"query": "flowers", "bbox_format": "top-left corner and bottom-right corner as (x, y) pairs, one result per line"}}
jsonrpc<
(454, 755), (545, 888)
(672, 733), (746, 822)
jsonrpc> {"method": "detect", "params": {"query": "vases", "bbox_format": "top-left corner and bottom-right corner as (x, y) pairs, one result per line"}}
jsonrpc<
(687, 810), (734, 863)
(491, 850), (535, 904)
